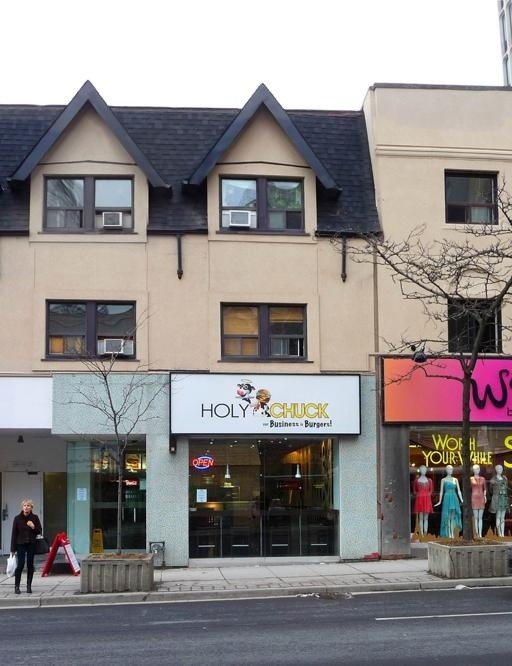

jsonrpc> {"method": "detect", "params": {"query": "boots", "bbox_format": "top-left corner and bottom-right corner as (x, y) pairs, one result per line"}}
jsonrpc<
(14, 571), (21, 594)
(27, 571), (33, 593)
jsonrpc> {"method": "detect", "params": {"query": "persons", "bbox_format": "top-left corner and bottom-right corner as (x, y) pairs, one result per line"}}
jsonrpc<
(10, 500), (42, 594)
(490, 465), (509, 538)
(412, 465), (433, 536)
(470, 465), (487, 538)
(433, 465), (464, 538)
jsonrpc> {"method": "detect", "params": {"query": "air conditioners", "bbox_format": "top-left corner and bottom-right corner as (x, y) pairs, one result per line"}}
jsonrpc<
(228, 209), (252, 228)
(103, 339), (124, 354)
(101, 209), (124, 229)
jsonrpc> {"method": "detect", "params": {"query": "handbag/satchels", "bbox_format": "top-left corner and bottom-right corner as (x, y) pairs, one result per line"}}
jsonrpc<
(34, 538), (49, 555)
(6, 552), (18, 577)
